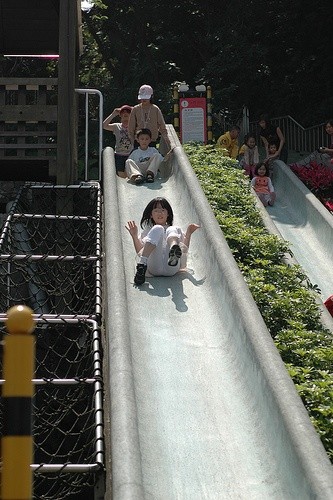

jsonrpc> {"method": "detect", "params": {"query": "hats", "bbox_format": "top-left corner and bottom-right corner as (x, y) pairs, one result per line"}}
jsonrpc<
(138, 85), (153, 100)
(121, 105), (133, 110)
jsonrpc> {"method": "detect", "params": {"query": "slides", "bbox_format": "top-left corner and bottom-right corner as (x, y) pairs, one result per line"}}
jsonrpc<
(101, 146), (333, 500)
(239, 159), (333, 334)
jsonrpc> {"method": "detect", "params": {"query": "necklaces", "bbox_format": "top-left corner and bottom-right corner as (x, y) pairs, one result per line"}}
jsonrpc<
(139, 103), (151, 128)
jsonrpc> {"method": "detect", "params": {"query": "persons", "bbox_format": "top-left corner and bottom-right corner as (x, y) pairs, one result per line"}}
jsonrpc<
(124, 129), (173, 183)
(321, 121), (333, 153)
(251, 163), (275, 206)
(103, 105), (134, 177)
(215, 125), (241, 160)
(128, 85), (168, 148)
(268, 142), (280, 159)
(239, 134), (259, 176)
(125, 197), (200, 285)
(257, 117), (288, 161)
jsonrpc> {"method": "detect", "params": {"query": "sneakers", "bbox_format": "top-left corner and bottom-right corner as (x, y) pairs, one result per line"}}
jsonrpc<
(167, 244), (182, 266)
(134, 175), (145, 186)
(133, 263), (148, 286)
(145, 172), (155, 183)
